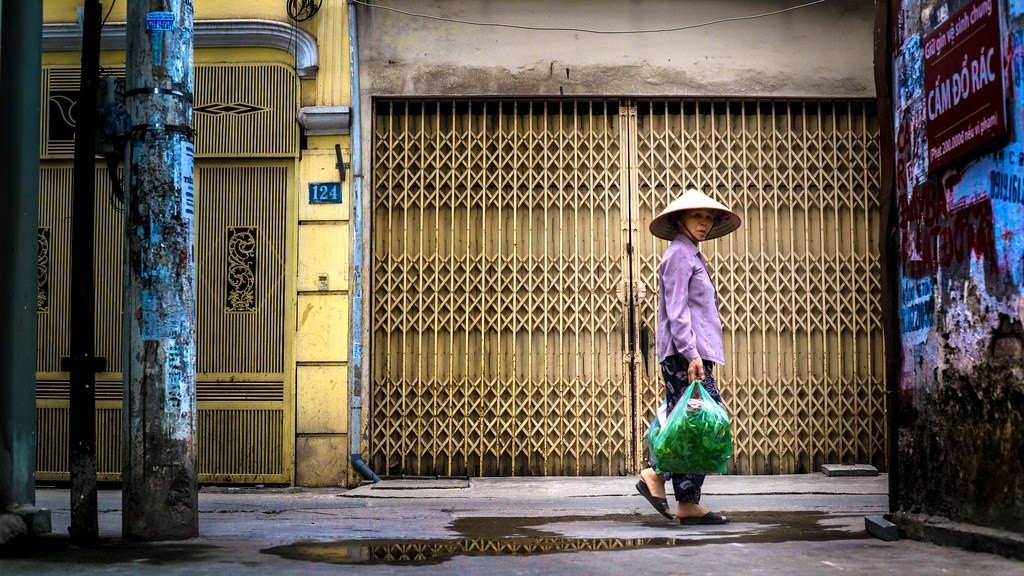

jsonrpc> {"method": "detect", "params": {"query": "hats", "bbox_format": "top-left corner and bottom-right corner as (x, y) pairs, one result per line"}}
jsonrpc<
(649, 188), (742, 241)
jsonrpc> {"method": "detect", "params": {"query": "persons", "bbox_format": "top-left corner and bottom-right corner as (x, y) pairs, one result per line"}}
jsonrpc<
(635, 188), (741, 524)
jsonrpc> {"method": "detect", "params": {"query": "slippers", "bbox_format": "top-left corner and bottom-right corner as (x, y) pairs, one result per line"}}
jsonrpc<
(679, 510), (728, 525)
(635, 480), (674, 520)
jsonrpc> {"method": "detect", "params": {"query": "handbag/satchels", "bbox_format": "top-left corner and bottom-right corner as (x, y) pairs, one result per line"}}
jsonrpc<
(646, 398), (673, 480)
(652, 379), (734, 475)
